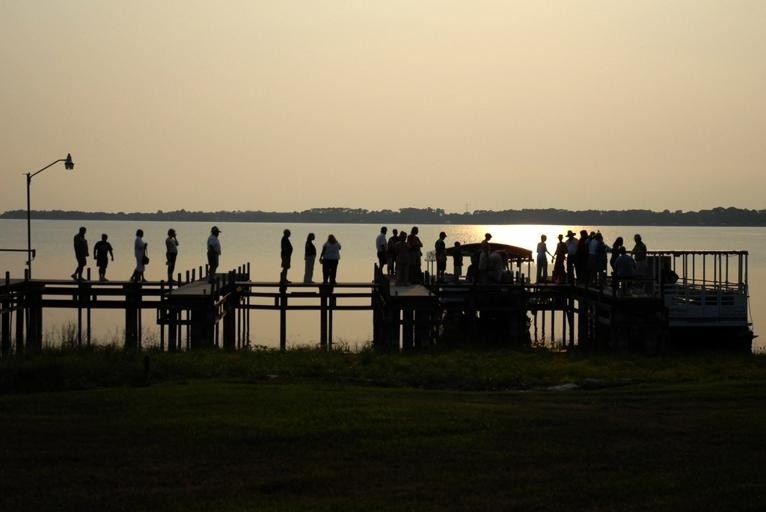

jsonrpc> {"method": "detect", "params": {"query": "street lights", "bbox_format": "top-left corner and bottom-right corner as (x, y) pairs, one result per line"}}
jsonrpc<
(26, 153), (74, 279)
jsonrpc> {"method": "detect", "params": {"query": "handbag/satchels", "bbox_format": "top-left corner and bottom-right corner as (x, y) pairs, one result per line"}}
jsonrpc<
(142, 255), (149, 265)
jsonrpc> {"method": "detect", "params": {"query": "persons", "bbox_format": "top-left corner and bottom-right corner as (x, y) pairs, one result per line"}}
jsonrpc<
(375, 226), (492, 288)
(206, 225), (223, 284)
(279, 226), (295, 285)
(317, 233), (343, 285)
(68, 227), (91, 283)
(303, 231), (318, 285)
(163, 226), (183, 283)
(91, 232), (114, 281)
(536, 229), (651, 296)
(128, 229), (148, 283)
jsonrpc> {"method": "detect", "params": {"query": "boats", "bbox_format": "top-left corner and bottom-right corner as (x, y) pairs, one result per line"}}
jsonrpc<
(427, 241), (532, 332)
(625, 247), (752, 340)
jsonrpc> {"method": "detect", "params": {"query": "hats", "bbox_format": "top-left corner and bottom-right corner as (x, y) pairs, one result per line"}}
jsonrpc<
(565, 230), (575, 236)
(212, 226), (221, 233)
